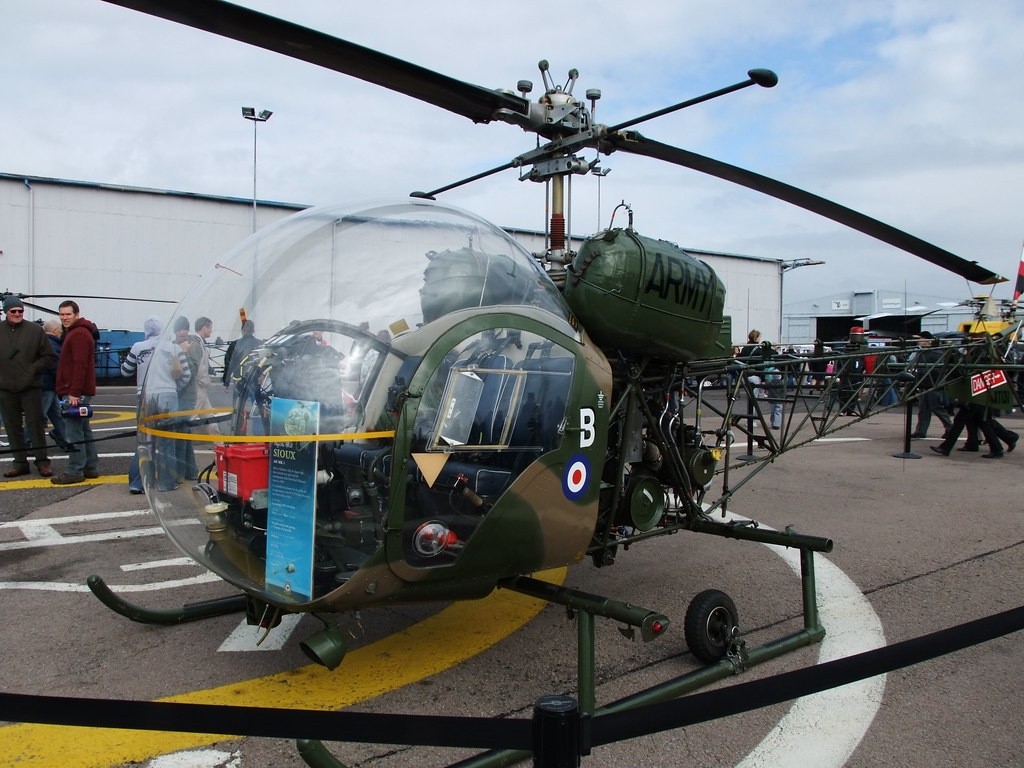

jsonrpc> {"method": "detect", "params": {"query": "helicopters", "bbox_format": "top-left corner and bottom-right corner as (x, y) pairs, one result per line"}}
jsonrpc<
(90, 1), (1023, 768)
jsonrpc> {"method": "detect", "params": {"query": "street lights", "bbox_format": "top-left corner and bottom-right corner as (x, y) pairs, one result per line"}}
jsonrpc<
(241, 106), (273, 317)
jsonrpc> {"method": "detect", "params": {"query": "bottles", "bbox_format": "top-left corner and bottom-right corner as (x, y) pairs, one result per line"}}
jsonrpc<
(60, 395), (90, 408)
(61, 404), (93, 419)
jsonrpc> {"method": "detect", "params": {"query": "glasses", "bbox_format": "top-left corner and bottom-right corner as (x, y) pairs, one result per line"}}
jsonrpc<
(10, 310), (23, 313)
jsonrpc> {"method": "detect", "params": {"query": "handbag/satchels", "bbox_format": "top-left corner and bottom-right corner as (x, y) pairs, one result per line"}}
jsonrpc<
(826, 360), (836, 375)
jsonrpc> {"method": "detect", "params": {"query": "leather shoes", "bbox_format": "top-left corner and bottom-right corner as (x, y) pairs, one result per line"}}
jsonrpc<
(930, 445), (949, 456)
(982, 451), (1003, 458)
(957, 446), (978, 452)
(1007, 434), (1019, 452)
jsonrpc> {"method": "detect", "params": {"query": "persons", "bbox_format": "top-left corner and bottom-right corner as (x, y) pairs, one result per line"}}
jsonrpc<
(727, 341), (898, 430)
(0, 295), (100, 484)
(740, 330), (765, 427)
(313, 330), (327, 346)
(222, 320), (268, 436)
(911, 331), (1019, 459)
(121, 316), (225, 494)
(359, 330), (391, 388)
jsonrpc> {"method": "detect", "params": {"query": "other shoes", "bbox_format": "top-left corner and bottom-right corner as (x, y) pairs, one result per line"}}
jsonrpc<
(847, 412), (856, 416)
(773, 426), (780, 430)
(910, 433), (926, 441)
(84, 471), (98, 478)
(3, 467), (31, 477)
(51, 472), (85, 484)
(38, 466), (53, 476)
(49, 431), (65, 449)
(129, 487), (142, 494)
(764, 393), (768, 398)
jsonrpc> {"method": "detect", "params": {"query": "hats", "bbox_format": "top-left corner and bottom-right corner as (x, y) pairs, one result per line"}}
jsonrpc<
(912, 331), (933, 339)
(3, 296), (24, 313)
